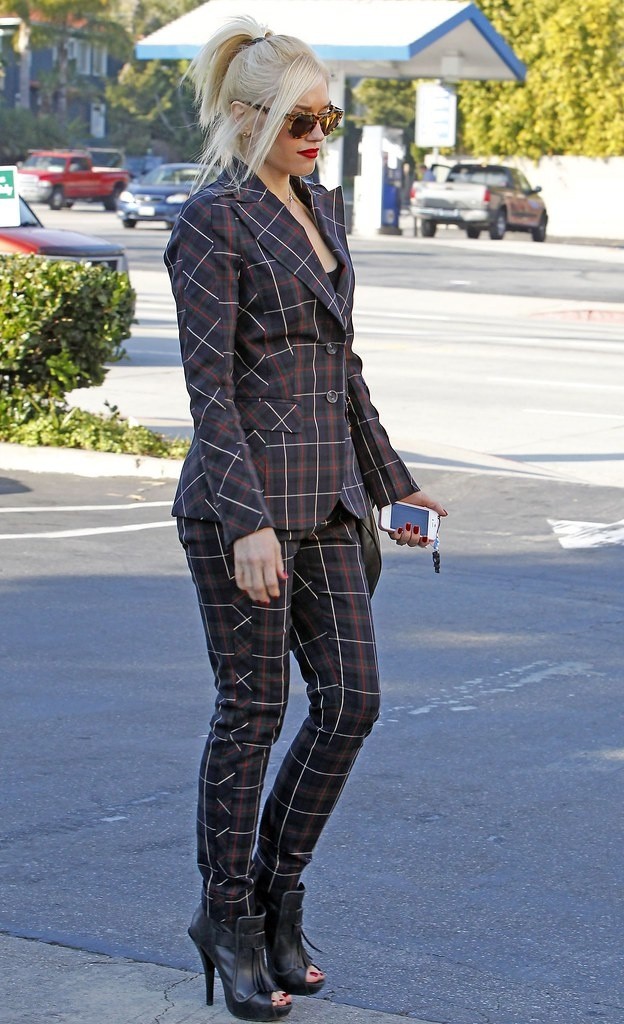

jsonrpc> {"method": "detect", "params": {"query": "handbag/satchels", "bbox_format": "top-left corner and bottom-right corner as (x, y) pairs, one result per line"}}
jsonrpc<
(346, 423), (382, 598)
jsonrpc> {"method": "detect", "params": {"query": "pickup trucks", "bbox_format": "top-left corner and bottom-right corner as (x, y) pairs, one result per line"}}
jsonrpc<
(8, 149), (129, 213)
(408, 159), (548, 242)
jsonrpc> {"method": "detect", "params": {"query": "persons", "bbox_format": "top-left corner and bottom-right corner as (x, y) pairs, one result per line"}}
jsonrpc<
(162, 15), (448, 1022)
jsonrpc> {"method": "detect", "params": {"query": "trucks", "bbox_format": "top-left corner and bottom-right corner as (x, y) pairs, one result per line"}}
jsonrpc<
(86, 146), (162, 177)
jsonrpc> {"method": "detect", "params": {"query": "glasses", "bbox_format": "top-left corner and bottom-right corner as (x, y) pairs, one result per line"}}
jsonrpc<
(231, 99), (343, 139)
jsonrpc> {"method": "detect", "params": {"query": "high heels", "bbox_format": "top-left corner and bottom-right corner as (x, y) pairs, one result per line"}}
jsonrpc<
(251, 882), (325, 996)
(188, 898), (292, 1022)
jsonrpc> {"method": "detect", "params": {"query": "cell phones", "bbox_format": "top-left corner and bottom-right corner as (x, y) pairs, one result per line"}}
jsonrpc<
(378, 501), (439, 541)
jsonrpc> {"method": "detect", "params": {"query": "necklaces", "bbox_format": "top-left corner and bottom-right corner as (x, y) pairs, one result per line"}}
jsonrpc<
(287, 187), (293, 213)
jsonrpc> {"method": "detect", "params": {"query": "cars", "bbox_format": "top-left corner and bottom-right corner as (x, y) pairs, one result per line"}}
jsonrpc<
(117, 163), (222, 230)
(0, 192), (129, 274)
